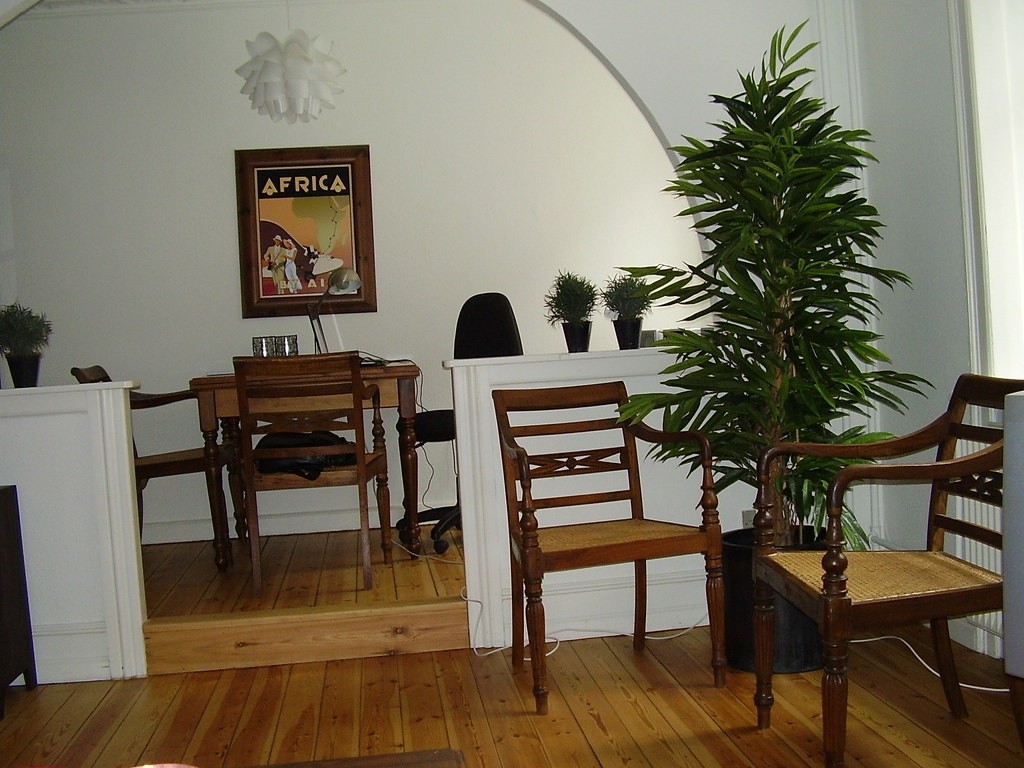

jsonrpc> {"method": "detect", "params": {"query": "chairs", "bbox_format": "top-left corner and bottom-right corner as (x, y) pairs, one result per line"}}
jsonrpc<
(70, 364), (249, 548)
(751, 372), (1024, 768)
(493, 380), (727, 716)
(396, 293), (525, 555)
(232, 349), (393, 598)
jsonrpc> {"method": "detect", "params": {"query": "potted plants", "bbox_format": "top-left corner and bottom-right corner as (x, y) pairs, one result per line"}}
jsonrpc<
(543, 270), (601, 353)
(599, 272), (654, 350)
(0, 303), (54, 389)
(614, 14), (937, 675)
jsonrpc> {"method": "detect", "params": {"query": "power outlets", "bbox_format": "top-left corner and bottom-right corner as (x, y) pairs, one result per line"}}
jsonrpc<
(742, 510), (756, 529)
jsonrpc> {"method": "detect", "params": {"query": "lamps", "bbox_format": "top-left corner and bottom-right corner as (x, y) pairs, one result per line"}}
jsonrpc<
(305, 265), (365, 354)
(234, 0), (347, 124)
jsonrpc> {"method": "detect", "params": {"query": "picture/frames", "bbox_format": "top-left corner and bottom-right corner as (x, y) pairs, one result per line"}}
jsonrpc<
(234, 144), (377, 319)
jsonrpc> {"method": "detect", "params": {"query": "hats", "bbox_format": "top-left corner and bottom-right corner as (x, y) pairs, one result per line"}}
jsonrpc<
(284, 239), (295, 248)
(273, 235), (282, 242)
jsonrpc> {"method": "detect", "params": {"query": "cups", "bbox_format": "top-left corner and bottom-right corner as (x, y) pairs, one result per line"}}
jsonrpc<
(276, 335), (298, 357)
(252, 336), (275, 357)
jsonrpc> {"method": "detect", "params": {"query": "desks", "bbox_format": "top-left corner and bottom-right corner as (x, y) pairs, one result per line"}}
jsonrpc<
(0, 485), (38, 720)
(188, 360), (421, 573)
